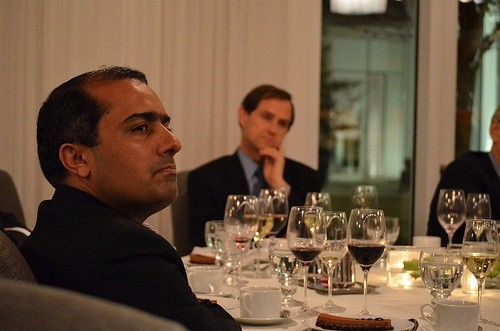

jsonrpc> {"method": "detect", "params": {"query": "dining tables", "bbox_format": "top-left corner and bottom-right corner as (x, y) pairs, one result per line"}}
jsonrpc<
(170, 229), (500, 331)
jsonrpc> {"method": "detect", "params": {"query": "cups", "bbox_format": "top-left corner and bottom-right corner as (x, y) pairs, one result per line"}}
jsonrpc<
(186, 265), (224, 294)
(420, 246), (465, 301)
(420, 296), (479, 331)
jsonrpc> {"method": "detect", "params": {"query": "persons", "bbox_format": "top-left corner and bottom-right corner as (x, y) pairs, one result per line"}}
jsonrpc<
(179, 83), (322, 255)
(426, 106), (500, 247)
(17, 68), (241, 331)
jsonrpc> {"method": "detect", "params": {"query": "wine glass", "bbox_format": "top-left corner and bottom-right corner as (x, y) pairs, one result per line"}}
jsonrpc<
(466, 193), (491, 241)
(207, 181), (401, 318)
(463, 218), (500, 323)
(437, 190), (466, 248)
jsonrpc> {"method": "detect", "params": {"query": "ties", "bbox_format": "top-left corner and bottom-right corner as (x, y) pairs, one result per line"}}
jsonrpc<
(252, 167), (270, 197)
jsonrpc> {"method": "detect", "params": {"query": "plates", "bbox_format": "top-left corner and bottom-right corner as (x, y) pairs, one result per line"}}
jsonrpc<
(232, 318), (288, 326)
(300, 317), (414, 331)
